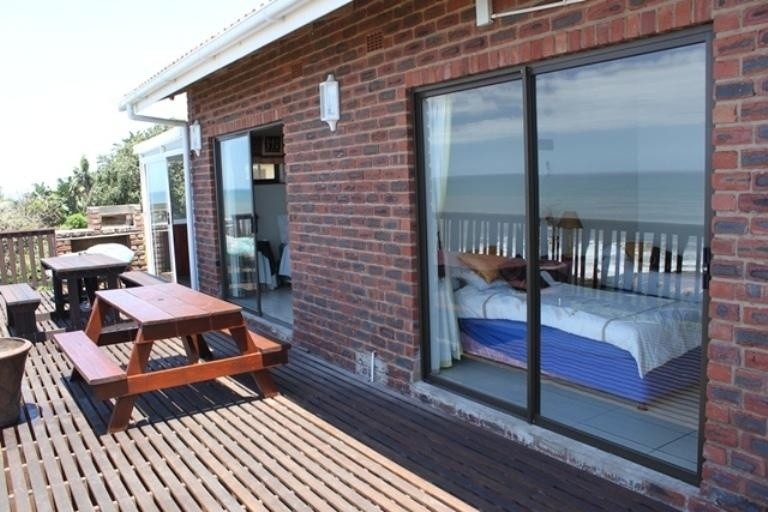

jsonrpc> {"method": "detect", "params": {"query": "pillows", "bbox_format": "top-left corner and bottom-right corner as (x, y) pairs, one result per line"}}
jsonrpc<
(450, 254), (534, 293)
(623, 240), (682, 273)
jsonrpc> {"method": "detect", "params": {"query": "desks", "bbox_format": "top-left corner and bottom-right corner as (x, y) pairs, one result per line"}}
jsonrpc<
(41, 254), (129, 326)
(94, 281), (278, 435)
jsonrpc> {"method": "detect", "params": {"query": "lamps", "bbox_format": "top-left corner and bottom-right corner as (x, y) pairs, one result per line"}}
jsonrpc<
(318, 76), (342, 132)
(188, 120), (204, 157)
(558, 209), (581, 255)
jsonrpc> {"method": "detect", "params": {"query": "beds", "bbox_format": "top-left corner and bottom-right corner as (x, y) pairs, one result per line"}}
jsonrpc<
(453, 251), (705, 409)
(584, 237), (708, 303)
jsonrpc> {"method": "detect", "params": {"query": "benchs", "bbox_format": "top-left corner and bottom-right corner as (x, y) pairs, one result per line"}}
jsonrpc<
(55, 328), (293, 434)
(120, 269), (164, 286)
(0, 281), (46, 341)
